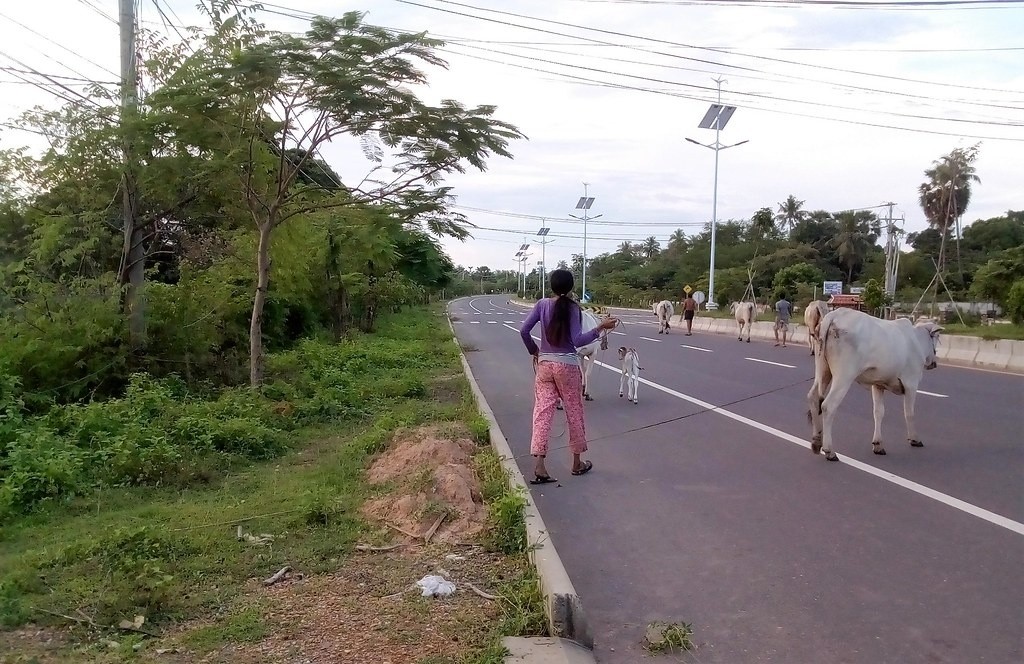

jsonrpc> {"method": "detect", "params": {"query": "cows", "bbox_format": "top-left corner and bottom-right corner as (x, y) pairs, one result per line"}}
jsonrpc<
(617, 346), (646, 404)
(555, 306), (610, 410)
(729, 301), (755, 343)
(649, 299), (674, 334)
(804, 300), (946, 462)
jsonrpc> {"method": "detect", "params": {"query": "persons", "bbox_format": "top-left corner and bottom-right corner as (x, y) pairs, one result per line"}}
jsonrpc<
(682, 294), (695, 336)
(520, 270), (617, 484)
(772, 293), (792, 347)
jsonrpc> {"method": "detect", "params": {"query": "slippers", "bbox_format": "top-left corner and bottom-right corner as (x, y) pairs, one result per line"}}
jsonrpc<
(530, 475), (558, 483)
(571, 460), (592, 475)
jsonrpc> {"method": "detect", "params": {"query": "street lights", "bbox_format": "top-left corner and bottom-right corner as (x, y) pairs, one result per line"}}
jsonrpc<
(568, 181), (604, 304)
(684, 75), (750, 311)
(512, 236), (534, 300)
(531, 217), (557, 299)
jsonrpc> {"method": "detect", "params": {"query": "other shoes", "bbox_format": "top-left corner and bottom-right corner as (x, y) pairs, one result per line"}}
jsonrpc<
(775, 343), (779, 347)
(783, 345), (786, 347)
(684, 332), (692, 336)
